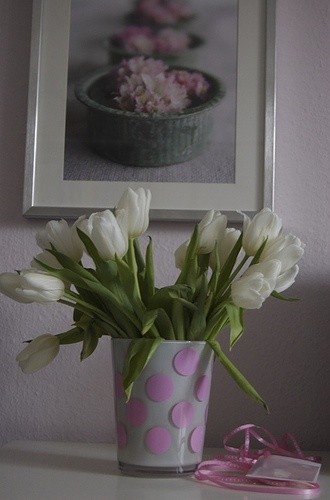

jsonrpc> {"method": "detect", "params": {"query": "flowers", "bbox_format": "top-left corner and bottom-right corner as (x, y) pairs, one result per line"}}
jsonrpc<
(0, 187), (306, 415)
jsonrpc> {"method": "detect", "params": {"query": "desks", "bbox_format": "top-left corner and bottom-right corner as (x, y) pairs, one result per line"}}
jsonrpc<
(0, 440), (330, 500)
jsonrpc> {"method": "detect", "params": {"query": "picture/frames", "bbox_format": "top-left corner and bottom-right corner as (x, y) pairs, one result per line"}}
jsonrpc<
(21, 0), (276, 225)
